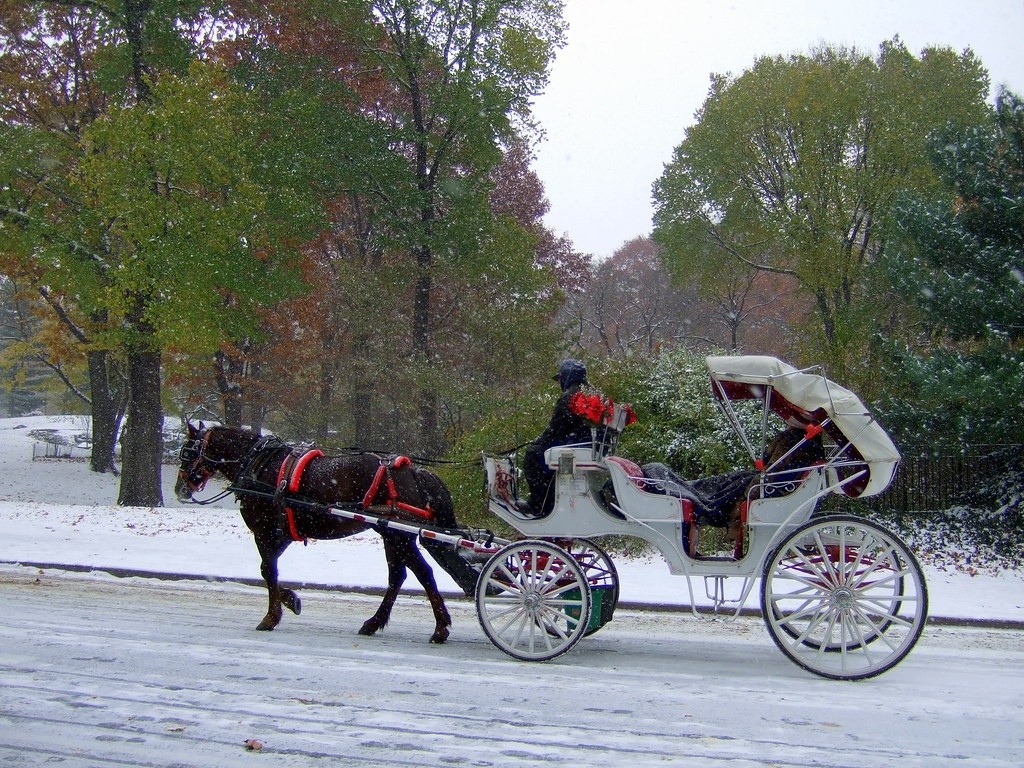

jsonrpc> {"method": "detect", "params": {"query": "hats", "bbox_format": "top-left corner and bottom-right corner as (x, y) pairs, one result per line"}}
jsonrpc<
(784, 412), (820, 430)
(551, 372), (560, 380)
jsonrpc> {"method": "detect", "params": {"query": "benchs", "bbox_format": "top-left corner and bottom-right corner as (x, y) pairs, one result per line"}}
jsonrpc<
(741, 460), (827, 528)
(546, 400), (628, 475)
(606, 456), (697, 523)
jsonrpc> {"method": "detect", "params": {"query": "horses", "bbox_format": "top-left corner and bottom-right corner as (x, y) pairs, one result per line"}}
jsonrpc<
(173, 420), (459, 645)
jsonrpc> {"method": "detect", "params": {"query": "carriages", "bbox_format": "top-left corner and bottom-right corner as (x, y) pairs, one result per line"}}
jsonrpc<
(174, 355), (928, 683)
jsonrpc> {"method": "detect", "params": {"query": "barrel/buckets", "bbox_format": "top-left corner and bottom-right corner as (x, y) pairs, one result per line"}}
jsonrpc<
(559, 585), (617, 633)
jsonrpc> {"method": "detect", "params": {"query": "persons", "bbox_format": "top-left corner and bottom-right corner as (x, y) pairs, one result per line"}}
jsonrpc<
(643, 410), (822, 527)
(516, 359), (606, 515)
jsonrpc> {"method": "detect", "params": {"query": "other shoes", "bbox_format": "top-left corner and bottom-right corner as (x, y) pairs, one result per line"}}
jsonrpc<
(516, 500), (543, 514)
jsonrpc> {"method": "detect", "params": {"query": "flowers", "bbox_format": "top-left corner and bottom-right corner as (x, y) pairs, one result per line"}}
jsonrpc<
(570, 391), (636, 431)
